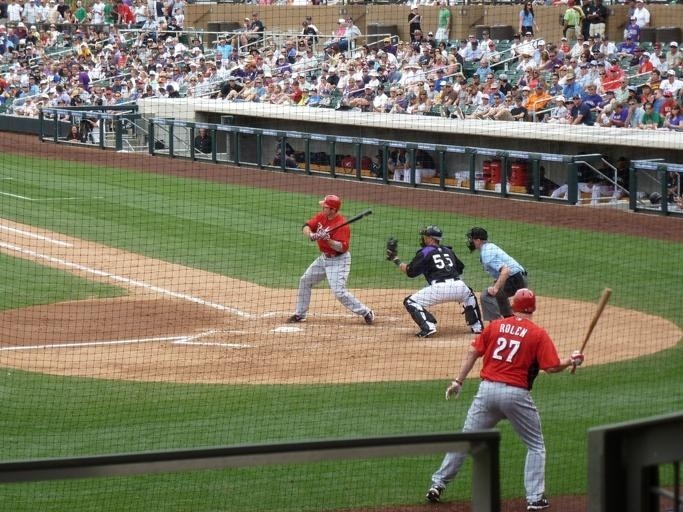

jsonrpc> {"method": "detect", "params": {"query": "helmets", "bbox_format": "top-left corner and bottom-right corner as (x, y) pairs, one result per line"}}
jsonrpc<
(649, 192), (662, 204)
(512, 288), (536, 313)
(318, 194), (341, 209)
(419, 225), (442, 247)
(465, 227), (487, 250)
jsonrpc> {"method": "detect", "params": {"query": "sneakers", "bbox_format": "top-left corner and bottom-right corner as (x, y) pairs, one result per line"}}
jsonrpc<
(365, 310), (374, 324)
(287, 314), (306, 322)
(527, 496), (551, 511)
(419, 328), (437, 338)
(426, 484), (443, 504)
(471, 327), (482, 334)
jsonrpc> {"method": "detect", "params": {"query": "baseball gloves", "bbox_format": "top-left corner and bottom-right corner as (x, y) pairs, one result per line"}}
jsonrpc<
(387, 237), (398, 261)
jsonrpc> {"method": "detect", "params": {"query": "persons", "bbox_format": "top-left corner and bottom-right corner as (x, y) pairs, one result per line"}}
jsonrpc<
(463, 227), (528, 324)
(194, 129), (212, 152)
(426, 288), (584, 509)
(285, 194), (374, 326)
(343, 148), (436, 183)
(215, 1), (682, 130)
(1, 0), (225, 143)
(666, 172), (682, 201)
(386, 226), (483, 336)
(526, 155), (633, 204)
(273, 137), (295, 168)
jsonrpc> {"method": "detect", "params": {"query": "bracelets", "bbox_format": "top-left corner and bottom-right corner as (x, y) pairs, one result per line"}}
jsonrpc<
(455, 378), (464, 387)
(394, 258), (400, 266)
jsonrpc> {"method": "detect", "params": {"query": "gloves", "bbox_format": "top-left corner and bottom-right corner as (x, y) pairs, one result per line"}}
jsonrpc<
(570, 350), (584, 366)
(319, 229), (327, 240)
(446, 381), (462, 400)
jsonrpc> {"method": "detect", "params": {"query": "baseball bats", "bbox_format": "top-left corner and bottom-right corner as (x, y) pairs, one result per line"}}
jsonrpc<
(569, 288), (612, 375)
(326, 209), (372, 233)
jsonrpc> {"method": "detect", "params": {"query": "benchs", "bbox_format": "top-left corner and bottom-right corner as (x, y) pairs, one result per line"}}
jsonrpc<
(581, 193), (629, 204)
(421, 177), (527, 193)
(298, 162), (373, 177)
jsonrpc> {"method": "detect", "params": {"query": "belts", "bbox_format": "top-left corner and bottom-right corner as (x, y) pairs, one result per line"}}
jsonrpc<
(429, 278), (460, 285)
(325, 251), (345, 258)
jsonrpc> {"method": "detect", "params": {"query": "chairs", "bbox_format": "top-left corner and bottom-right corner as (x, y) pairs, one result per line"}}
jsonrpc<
(0, 22), (683, 132)
